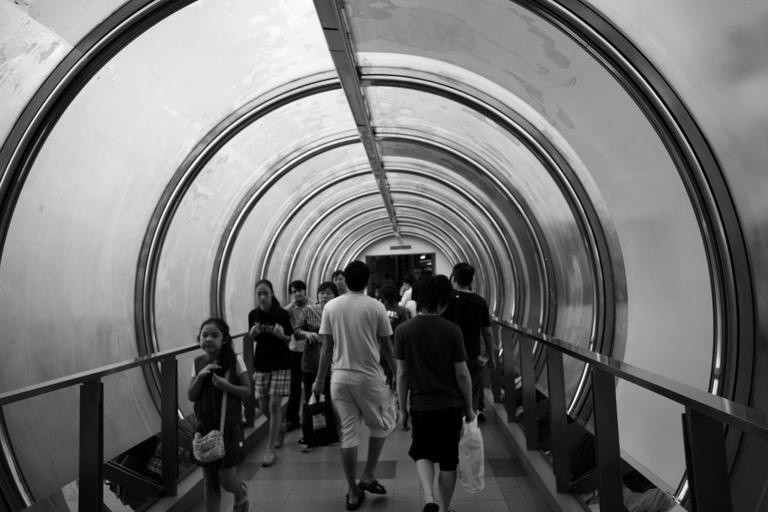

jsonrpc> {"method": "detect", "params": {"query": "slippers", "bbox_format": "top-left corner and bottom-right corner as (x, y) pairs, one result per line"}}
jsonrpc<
(346, 488), (365, 510)
(357, 479), (387, 495)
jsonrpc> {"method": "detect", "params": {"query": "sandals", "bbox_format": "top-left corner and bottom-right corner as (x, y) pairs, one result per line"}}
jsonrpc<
(232, 483), (248, 511)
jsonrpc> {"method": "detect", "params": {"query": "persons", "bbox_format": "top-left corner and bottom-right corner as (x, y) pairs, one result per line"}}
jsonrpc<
(439, 261), (498, 422)
(394, 274), (478, 512)
(293, 281), (339, 452)
(282, 277), (316, 432)
(311, 258), (397, 512)
(314, 270), (434, 328)
(246, 279), (293, 467)
(187, 318), (252, 512)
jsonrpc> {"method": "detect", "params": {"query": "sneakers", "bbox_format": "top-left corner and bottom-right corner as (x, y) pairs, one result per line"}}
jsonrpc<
(261, 450), (277, 467)
(275, 423), (286, 449)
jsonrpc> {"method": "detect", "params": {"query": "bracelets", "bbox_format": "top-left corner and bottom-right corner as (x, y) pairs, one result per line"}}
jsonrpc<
(314, 376), (325, 383)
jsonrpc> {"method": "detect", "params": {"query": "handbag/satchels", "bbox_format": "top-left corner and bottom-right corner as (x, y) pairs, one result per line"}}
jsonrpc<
(302, 398), (341, 449)
(457, 414), (484, 494)
(193, 430), (226, 464)
